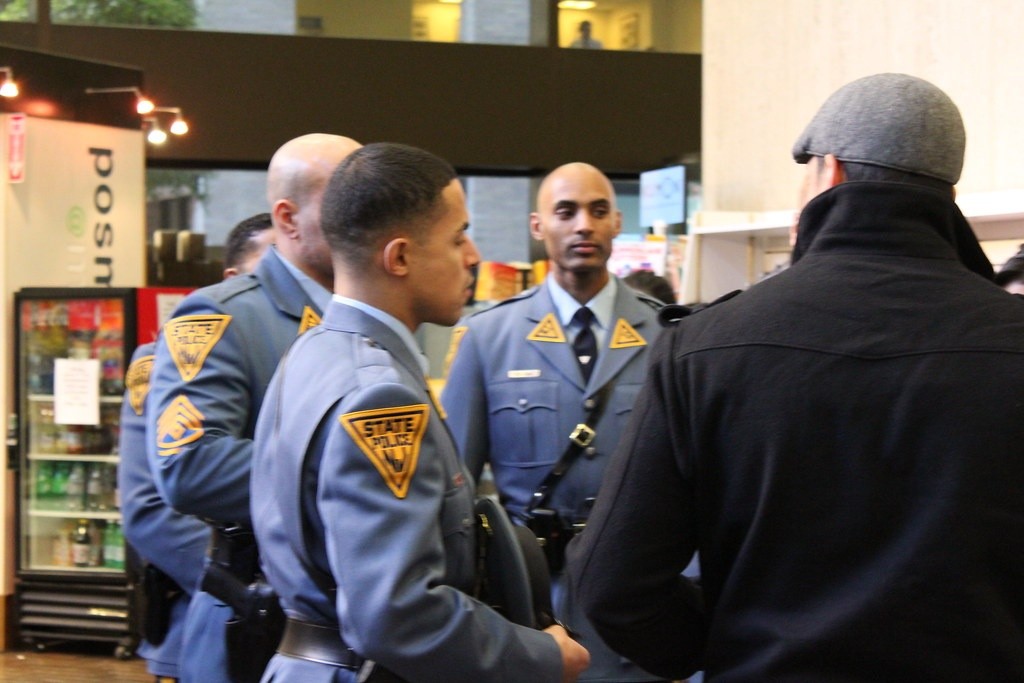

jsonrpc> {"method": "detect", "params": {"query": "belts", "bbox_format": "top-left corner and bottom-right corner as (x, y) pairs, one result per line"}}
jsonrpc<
(276, 619), (362, 670)
(207, 527), (260, 572)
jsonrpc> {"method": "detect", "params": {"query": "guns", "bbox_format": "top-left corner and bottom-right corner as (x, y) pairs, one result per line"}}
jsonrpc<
(199, 520), (252, 616)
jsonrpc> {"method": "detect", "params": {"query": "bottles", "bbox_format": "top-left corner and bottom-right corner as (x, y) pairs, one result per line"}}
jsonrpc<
(24, 326), (126, 569)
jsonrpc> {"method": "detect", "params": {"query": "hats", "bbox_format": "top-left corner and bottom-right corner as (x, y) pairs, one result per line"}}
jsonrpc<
(792, 73), (966, 184)
(476, 497), (551, 631)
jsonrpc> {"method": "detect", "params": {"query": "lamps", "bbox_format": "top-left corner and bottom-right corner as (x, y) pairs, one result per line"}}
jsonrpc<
(0, 66), (19, 98)
(143, 116), (166, 145)
(75, 79), (153, 113)
(149, 100), (190, 135)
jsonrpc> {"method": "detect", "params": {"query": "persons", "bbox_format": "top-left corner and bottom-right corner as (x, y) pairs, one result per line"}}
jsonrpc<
(145, 133), (364, 683)
(623, 270), (676, 305)
(440, 162), (703, 683)
(257, 144), (590, 683)
(119, 212), (277, 683)
(565, 72), (1024, 683)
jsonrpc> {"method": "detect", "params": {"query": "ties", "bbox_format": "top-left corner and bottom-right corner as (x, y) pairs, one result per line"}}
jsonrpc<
(572, 307), (599, 384)
